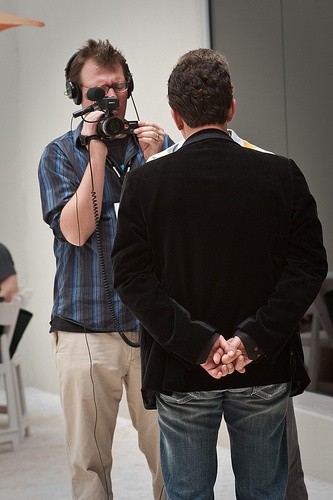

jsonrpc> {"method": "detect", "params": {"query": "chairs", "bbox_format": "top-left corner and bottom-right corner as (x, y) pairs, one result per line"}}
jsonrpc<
(0, 289), (32, 449)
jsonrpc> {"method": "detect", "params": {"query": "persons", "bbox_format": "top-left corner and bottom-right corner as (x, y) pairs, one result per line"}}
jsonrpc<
(0, 241), (19, 363)
(36, 38), (179, 499)
(111, 47), (330, 500)
(143, 81), (308, 500)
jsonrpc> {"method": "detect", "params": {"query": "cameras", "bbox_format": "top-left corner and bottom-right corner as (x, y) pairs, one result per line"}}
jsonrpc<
(72, 97), (138, 142)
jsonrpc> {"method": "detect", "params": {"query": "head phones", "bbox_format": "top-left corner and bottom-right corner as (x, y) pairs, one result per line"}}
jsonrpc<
(65, 52), (134, 105)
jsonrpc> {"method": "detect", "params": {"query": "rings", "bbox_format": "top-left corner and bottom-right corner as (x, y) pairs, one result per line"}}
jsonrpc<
(156, 133), (160, 141)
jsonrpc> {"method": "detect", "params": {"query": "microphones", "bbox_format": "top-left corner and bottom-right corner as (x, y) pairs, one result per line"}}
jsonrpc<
(87, 87), (105, 101)
